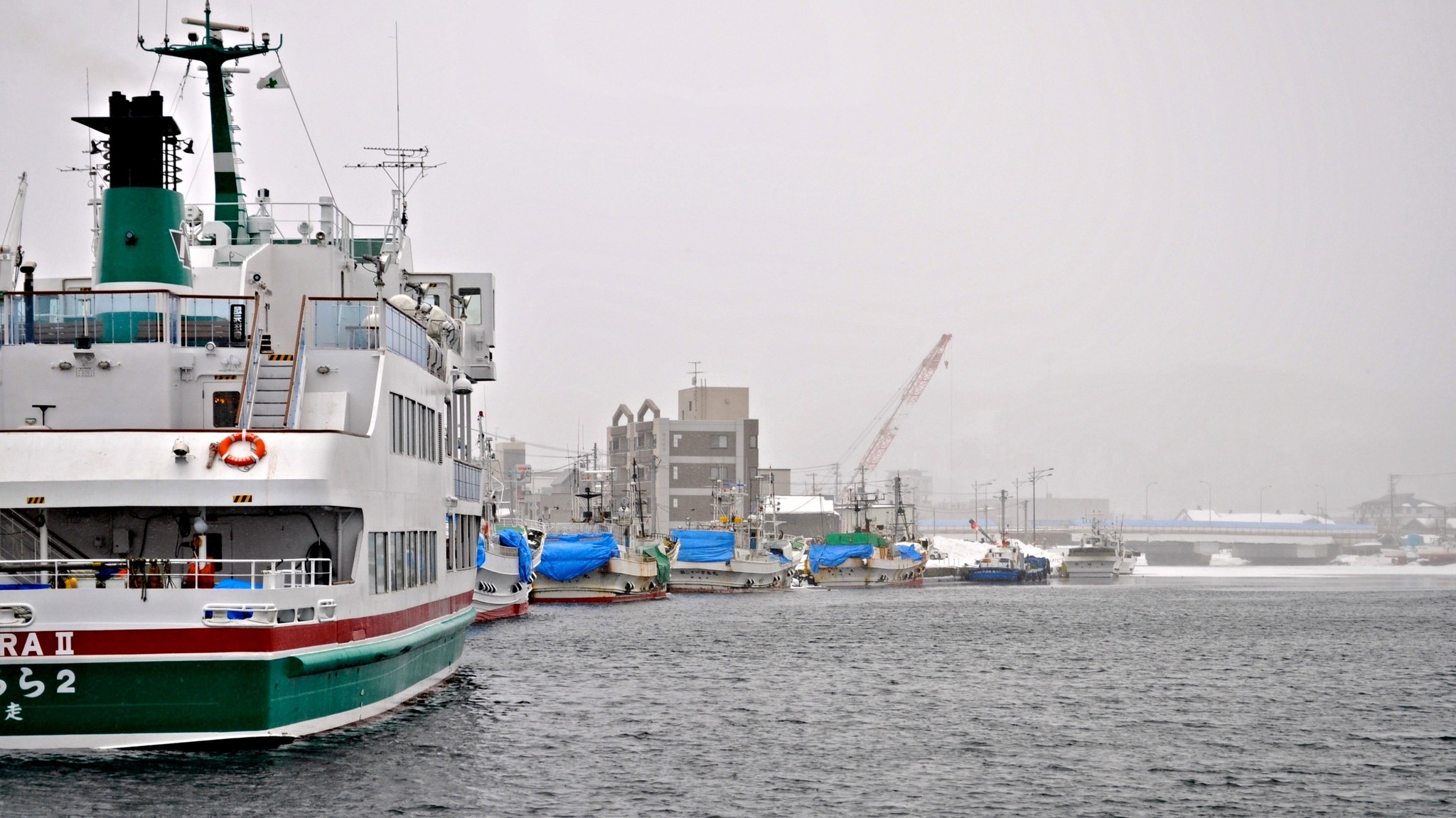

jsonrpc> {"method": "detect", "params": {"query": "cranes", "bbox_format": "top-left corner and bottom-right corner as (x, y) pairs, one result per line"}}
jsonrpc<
(808, 332), (959, 532)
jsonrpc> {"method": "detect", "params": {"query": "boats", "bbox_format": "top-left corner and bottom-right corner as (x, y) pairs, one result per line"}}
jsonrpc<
(445, 409), (548, 619)
(1380, 541), (1456, 566)
(0, 0), (496, 760)
(1051, 546), (1135, 575)
(664, 467), (811, 594)
(525, 456), (682, 606)
(804, 496), (930, 587)
(1061, 512), (1126, 577)
(959, 519), (1053, 583)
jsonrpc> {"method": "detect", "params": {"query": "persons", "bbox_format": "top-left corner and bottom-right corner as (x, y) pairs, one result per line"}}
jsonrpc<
(184, 533), (215, 588)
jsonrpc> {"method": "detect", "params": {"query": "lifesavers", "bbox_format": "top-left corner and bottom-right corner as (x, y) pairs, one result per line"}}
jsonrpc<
(484, 521), (489, 539)
(218, 432), (265, 466)
